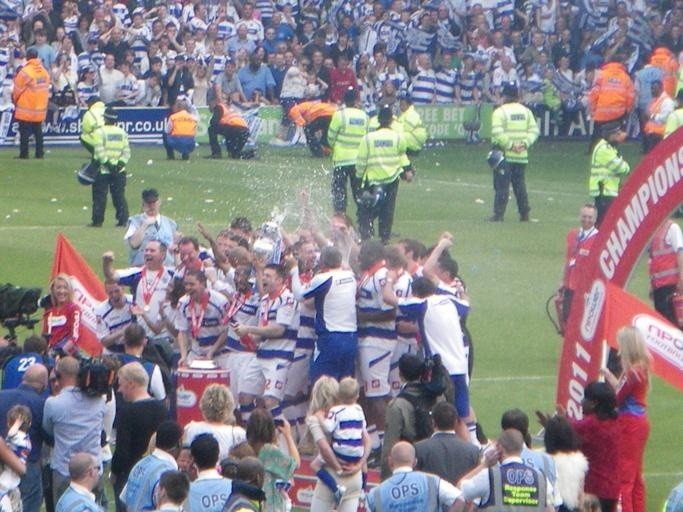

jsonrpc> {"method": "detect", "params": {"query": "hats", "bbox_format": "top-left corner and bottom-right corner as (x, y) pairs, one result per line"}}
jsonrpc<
(502, 81), (518, 98)
(101, 107), (119, 120)
(143, 189), (159, 203)
(85, 96), (100, 104)
(602, 121), (621, 136)
(378, 104), (393, 123)
(344, 85), (357, 100)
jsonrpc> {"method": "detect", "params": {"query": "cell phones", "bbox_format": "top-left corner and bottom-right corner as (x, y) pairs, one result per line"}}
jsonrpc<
(273, 419), (284, 428)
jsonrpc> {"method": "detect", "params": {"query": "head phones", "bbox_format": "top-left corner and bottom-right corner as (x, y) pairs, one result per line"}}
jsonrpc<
(216, 459), (238, 474)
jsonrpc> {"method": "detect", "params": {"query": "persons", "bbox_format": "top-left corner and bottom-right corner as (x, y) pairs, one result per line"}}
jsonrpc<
(1, 190), (483, 512)
(564, 204), (600, 322)
(327, 88), (427, 242)
(647, 220), (683, 327)
(484, 85), (541, 222)
(87, 108), (130, 228)
(588, 122), (630, 222)
(455, 326), (652, 510)
(1, 0), (683, 159)
